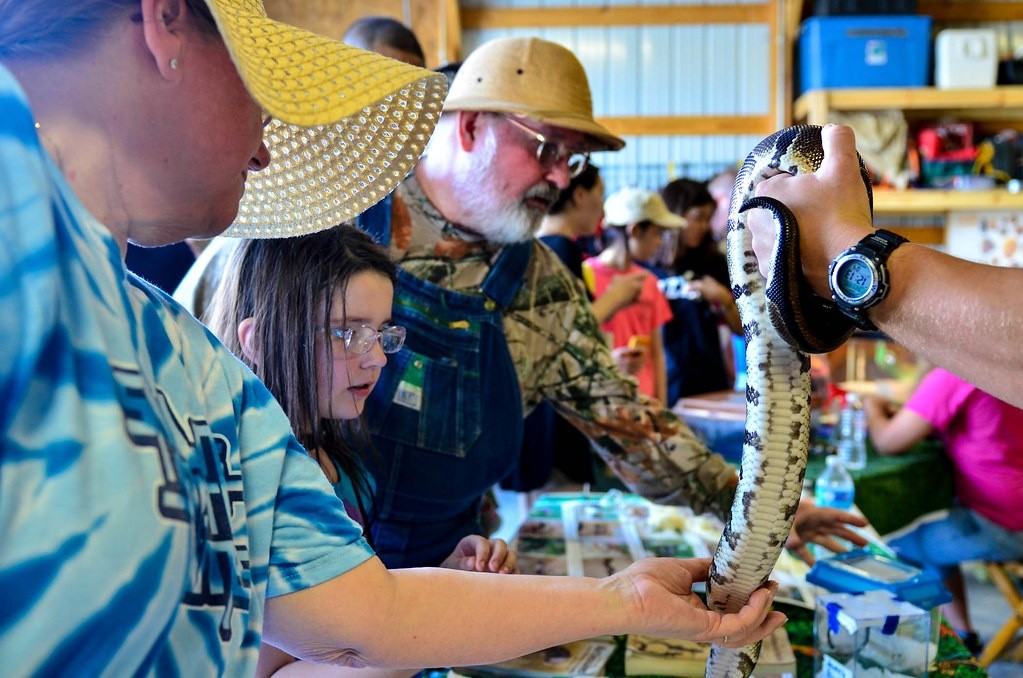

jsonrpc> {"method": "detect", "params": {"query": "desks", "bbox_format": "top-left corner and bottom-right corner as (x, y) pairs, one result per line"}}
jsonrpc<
(668, 388), (752, 469)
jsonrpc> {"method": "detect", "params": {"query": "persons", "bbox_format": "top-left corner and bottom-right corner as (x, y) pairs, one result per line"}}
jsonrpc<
(745, 121), (1023, 409)
(199, 223), (522, 678)
(323, 16), (871, 572)
(860, 364), (1023, 655)
(0, 0), (789, 678)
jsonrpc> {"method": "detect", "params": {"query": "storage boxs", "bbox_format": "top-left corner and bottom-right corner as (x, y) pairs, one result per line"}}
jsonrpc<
(800, 12), (933, 96)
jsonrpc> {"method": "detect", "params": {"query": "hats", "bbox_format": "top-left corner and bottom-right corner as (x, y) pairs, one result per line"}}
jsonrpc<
(204, 0), (448, 242)
(602, 189), (687, 230)
(442, 36), (625, 154)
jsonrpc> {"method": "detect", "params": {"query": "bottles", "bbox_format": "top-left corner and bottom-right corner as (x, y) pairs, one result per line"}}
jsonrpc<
(838, 391), (867, 472)
(815, 455), (854, 564)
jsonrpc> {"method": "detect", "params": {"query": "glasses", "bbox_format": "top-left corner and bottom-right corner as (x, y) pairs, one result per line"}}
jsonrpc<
(497, 112), (590, 180)
(129, 0), (273, 130)
(314, 322), (406, 353)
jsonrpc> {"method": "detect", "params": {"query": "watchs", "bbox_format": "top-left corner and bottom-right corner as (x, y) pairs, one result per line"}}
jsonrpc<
(827, 222), (910, 333)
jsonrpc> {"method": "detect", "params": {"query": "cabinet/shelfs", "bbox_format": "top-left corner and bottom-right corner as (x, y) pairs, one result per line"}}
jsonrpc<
(773, 0), (1023, 208)
